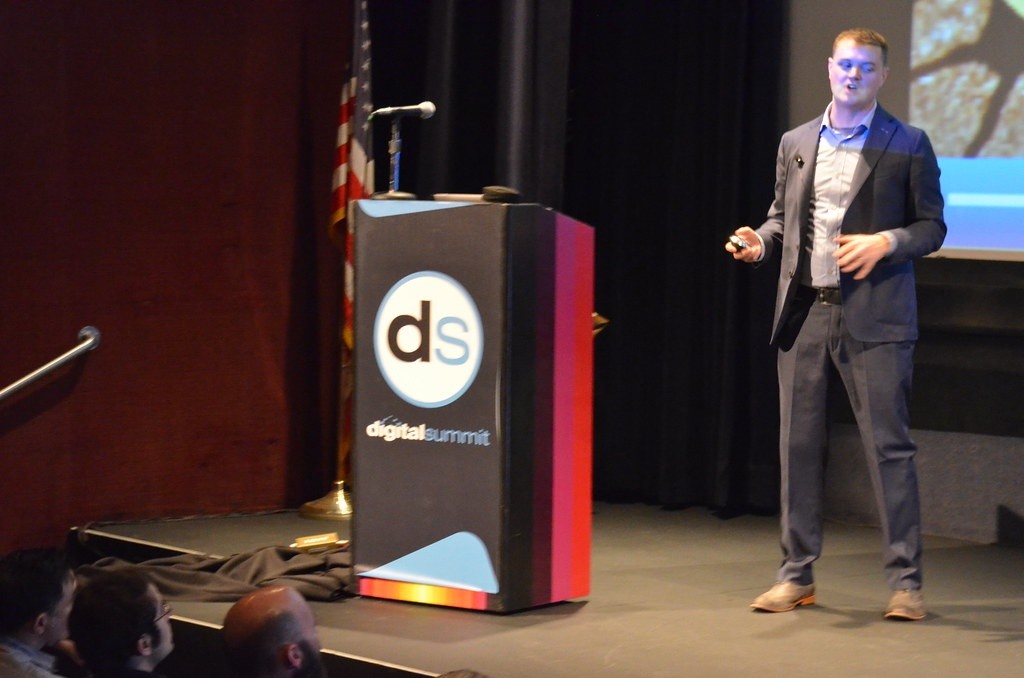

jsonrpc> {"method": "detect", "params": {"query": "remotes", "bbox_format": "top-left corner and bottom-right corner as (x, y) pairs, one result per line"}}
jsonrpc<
(729, 235), (752, 253)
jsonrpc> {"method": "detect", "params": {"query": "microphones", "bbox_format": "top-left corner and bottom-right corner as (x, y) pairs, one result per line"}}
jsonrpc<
(795, 154), (803, 164)
(376, 101), (436, 119)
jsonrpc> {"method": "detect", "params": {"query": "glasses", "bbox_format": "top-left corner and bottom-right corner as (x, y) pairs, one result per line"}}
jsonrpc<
(140, 602), (172, 636)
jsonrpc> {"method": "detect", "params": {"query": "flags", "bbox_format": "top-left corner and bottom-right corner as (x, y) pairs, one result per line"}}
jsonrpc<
(328, 0), (376, 485)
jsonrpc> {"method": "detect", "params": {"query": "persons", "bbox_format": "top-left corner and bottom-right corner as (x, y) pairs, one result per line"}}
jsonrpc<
(69, 567), (176, 678)
(724, 27), (949, 620)
(0, 545), (74, 678)
(221, 584), (326, 678)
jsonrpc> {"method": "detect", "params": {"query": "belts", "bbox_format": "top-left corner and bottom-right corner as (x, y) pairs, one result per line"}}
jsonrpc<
(796, 283), (842, 306)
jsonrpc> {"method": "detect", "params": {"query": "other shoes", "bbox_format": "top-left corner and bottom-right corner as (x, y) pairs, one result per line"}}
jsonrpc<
(750, 580), (816, 611)
(884, 588), (926, 620)
